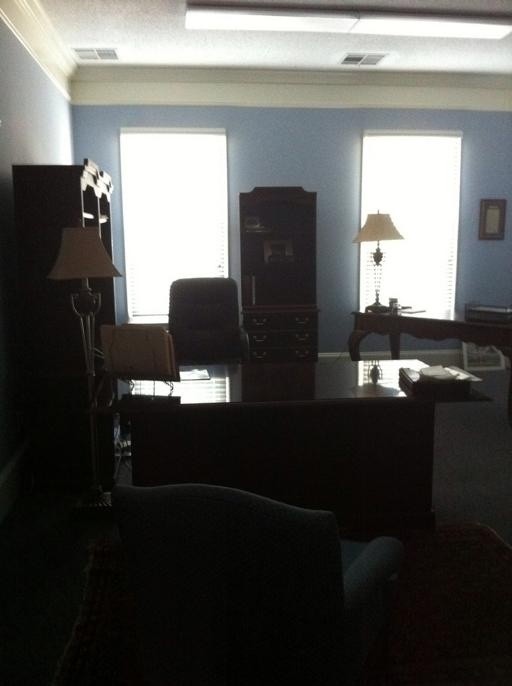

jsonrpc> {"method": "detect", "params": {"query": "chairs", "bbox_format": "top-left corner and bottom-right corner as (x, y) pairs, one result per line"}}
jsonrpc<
(110, 484), (405, 684)
(168, 276), (241, 361)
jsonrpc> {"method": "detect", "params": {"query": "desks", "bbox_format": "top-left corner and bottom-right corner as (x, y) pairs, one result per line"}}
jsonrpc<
(345, 307), (511, 426)
(105, 358), (493, 538)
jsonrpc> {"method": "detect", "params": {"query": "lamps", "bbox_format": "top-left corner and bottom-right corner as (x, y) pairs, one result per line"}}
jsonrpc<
(45, 225), (118, 512)
(99, 321), (181, 412)
(351, 210), (405, 311)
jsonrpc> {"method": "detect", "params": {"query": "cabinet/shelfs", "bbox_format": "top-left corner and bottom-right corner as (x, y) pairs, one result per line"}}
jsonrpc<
(238, 185), (321, 362)
(11, 158), (118, 500)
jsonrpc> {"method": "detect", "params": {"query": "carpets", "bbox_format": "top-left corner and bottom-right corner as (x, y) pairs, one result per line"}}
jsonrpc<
(53, 519), (510, 685)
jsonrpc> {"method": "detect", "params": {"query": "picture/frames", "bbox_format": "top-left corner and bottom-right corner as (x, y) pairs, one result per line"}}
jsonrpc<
(479, 198), (507, 239)
